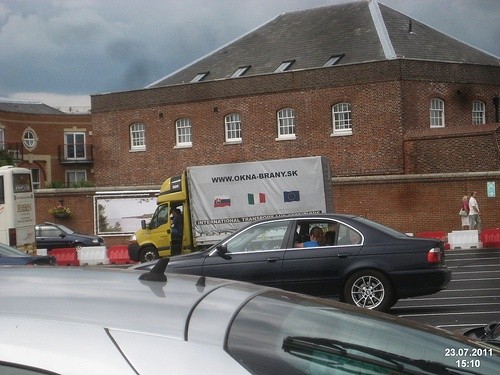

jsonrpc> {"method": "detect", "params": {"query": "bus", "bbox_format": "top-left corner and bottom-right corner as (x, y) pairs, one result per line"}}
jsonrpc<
(0, 165), (38, 255)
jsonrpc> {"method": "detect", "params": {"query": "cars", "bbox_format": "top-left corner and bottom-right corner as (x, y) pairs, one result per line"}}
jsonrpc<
(0, 264), (500, 375)
(127, 213), (453, 313)
(0, 243), (57, 267)
(34, 221), (104, 249)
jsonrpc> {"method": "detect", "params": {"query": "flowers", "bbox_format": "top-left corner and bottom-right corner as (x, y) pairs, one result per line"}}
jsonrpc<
(47, 206), (71, 214)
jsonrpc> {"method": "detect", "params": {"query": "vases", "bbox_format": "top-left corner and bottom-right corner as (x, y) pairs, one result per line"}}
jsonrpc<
(54, 213), (69, 218)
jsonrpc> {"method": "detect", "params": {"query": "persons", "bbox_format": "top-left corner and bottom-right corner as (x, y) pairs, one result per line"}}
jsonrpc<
(164, 209), (183, 233)
(461, 191), (481, 235)
(294, 227), (324, 248)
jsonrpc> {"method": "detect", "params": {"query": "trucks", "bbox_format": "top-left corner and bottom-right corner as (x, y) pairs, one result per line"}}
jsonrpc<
(125, 155), (336, 265)
